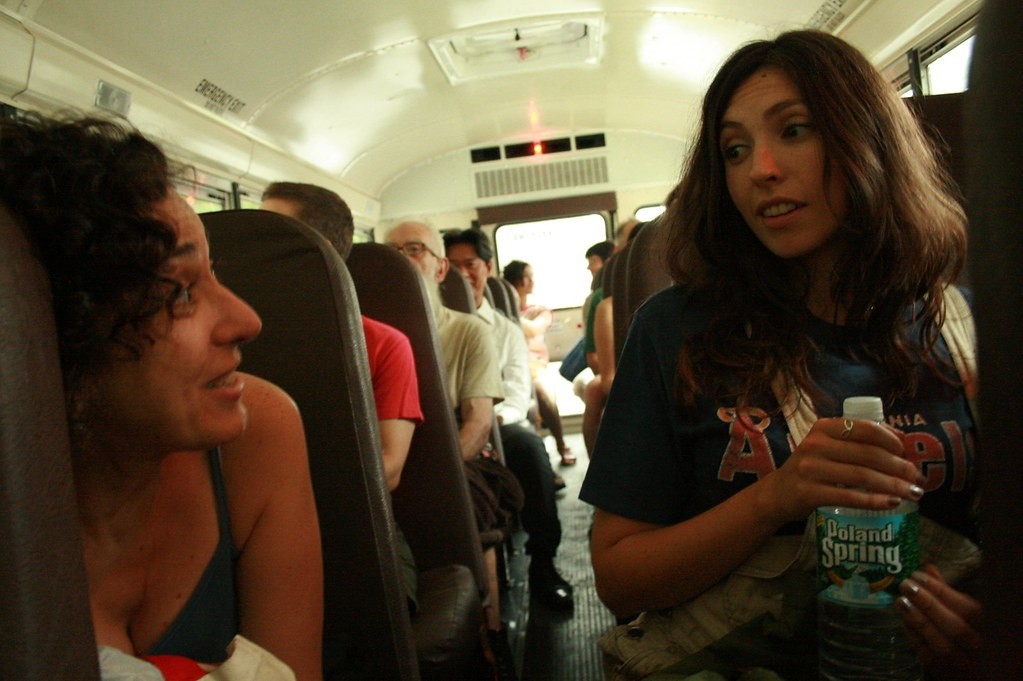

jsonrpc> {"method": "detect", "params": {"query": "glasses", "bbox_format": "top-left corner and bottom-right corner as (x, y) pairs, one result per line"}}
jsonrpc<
(385, 240), (442, 263)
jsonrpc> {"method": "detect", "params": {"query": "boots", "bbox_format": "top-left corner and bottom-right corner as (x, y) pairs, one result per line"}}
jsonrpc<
(487, 621), (517, 681)
(529, 554), (573, 612)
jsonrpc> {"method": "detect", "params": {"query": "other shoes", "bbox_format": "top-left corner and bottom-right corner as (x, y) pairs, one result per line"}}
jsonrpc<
(552, 471), (566, 491)
(558, 445), (576, 465)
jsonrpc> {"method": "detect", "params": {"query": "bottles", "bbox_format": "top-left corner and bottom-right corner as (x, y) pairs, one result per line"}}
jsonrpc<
(817, 397), (914, 681)
(501, 530), (531, 636)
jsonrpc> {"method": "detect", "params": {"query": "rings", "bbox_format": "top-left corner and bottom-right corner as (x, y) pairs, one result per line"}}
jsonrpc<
(840, 418), (855, 439)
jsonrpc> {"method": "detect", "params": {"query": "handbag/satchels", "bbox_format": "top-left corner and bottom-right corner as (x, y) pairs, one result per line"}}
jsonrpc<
(560, 339), (587, 382)
(598, 506), (983, 681)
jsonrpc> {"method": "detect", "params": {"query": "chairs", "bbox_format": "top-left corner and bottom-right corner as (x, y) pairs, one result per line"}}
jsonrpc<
(593, 0), (1022, 681)
(1, 206), (538, 680)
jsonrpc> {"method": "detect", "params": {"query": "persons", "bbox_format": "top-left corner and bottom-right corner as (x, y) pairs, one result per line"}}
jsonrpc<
(0, 113), (325, 681)
(579, 30), (1023, 681)
(260, 182), (647, 681)
